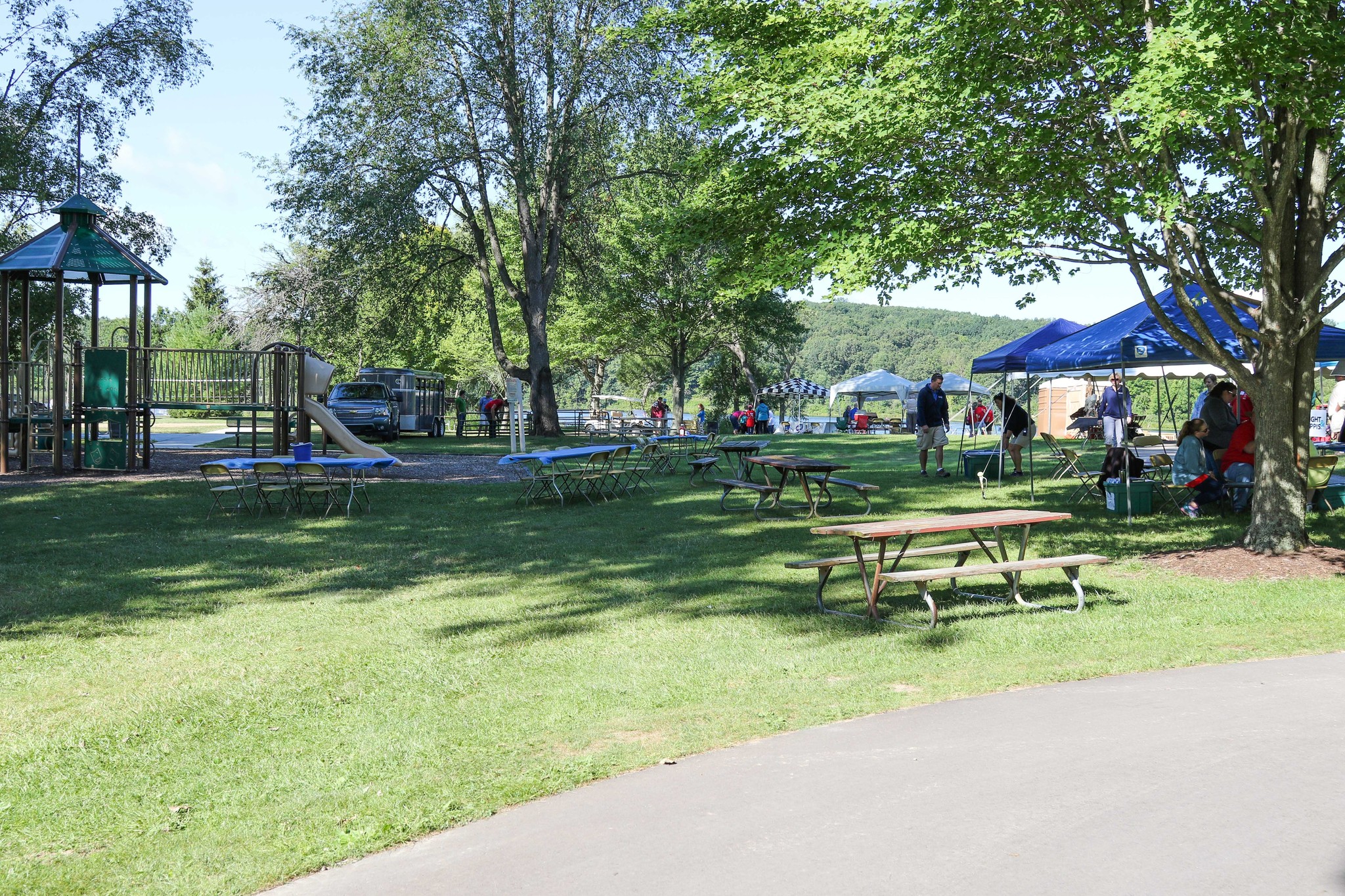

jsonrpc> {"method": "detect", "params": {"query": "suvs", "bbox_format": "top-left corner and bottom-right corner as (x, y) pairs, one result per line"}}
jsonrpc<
(316, 381), (401, 442)
(0, 393), (46, 411)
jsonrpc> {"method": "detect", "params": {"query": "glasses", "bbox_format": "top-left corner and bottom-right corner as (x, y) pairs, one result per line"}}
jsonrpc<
(1111, 378), (1119, 381)
(463, 394), (466, 395)
(1228, 391), (1236, 397)
(1195, 428), (1208, 433)
(932, 374), (942, 380)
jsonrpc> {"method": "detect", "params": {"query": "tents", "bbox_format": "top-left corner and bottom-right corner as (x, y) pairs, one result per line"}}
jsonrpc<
(957, 318), (1177, 503)
(829, 369), (916, 433)
(753, 377), (840, 434)
(1025, 282), (1345, 527)
(900, 373), (998, 435)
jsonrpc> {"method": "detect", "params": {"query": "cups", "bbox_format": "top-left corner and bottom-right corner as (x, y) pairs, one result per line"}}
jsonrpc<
(1118, 469), (1126, 483)
(1330, 439), (1338, 446)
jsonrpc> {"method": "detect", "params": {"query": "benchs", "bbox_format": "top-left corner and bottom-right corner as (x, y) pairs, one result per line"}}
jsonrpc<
(688, 458), (719, 475)
(612, 427), (677, 434)
(784, 541), (997, 569)
(877, 554), (1108, 583)
(714, 479), (782, 514)
(807, 475), (879, 504)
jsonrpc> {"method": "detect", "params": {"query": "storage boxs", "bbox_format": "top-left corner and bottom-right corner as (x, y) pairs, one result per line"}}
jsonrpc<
(1316, 474), (1345, 510)
(1103, 481), (1154, 516)
(962, 449), (1006, 479)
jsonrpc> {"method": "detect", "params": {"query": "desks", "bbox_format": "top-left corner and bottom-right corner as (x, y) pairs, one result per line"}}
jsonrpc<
(742, 455), (850, 517)
(614, 417), (675, 442)
(773, 421), (827, 434)
(811, 509), (1072, 621)
(498, 444), (637, 507)
(650, 435), (708, 476)
(713, 441), (771, 480)
(851, 420), (906, 434)
(204, 458), (397, 518)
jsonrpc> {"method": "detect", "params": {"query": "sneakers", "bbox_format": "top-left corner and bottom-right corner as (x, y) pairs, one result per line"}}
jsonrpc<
(921, 469), (928, 477)
(1180, 502), (1203, 518)
(936, 468), (950, 477)
(1009, 468), (1024, 477)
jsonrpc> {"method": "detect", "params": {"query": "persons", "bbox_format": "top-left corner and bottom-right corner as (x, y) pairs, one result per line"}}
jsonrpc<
(697, 404), (705, 436)
(730, 400), (775, 436)
(993, 392), (1036, 477)
(478, 390), (494, 437)
(1098, 372), (1133, 452)
(917, 373), (951, 478)
(1171, 374), (1345, 520)
(650, 397), (671, 436)
(968, 399), (994, 438)
(484, 399), (509, 438)
(902, 394), (917, 434)
(455, 390), (467, 437)
(843, 403), (859, 425)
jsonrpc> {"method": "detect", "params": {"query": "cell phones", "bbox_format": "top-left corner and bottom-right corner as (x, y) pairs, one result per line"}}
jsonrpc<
(1023, 428), (1027, 436)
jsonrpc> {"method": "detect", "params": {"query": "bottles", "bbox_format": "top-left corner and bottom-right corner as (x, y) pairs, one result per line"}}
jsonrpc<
(679, 424), (686, 436)
(1106, 478), (1120, 484)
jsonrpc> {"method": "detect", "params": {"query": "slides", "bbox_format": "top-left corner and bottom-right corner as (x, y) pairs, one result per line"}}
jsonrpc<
(304, 397), (402, 468)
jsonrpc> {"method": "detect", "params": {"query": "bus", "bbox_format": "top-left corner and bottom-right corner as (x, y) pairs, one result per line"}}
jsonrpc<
(358, 367), (446, 438)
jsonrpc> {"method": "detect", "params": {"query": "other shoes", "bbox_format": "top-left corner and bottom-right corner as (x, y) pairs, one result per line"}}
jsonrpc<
(1306, 503), (1313, 514)
(456, 434), (465, 438)
(1246, 505), (1252, 509)
(1229, 506), (1244, 513)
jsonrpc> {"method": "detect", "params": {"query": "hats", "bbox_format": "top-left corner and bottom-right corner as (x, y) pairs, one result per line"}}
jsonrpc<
(972, 402), (977, 406)
(749, 405), (752, 407)
(978, 399), (982, 402)
(663, 399), (666, 402)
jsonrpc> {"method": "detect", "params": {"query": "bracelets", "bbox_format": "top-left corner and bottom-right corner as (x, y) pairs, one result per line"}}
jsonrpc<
(1006, 436), (1010, 439)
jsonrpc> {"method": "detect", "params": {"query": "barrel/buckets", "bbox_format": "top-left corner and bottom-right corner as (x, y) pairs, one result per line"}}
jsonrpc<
(290, 443), (314, 461)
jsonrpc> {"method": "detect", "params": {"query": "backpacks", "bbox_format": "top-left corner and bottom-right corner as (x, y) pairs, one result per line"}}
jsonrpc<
(966, 417), (969, 425)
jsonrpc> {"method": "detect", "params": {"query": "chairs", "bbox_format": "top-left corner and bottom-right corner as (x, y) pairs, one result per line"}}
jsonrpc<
(199, 432), (721, 518)
(1061, 448), (1106, 506)
(835, 417), (902, 434)
(781, 422), (790, 433)
(1096, 435), (1338, 515)
(811, 423), (820, 429)
(1040, 433), (1082, 481)
